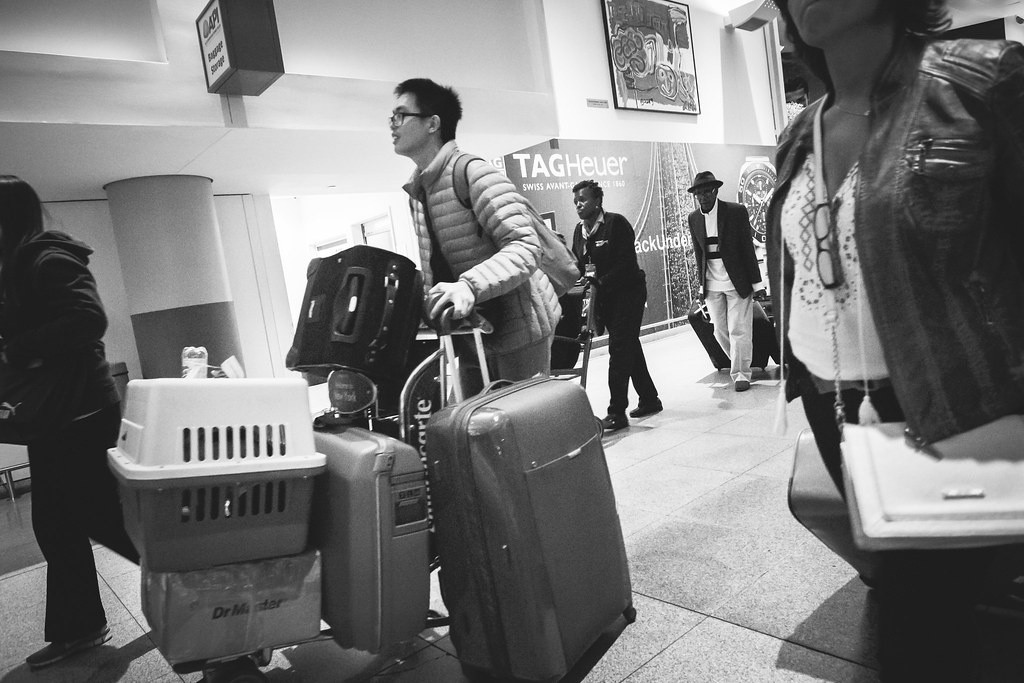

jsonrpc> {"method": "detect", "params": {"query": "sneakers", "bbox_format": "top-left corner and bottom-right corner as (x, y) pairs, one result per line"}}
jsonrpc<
(602, 412), (629, 430)
(26, 628), (105, 670)
(631, 399), (663, 421)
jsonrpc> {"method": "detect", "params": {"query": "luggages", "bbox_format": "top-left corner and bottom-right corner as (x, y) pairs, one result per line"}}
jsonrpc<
(786, 428), (871, 589)
(425, 302), (636, 683)
(314, 414), (431, 658)
(687, 295), (768, 370)
(286, 244), (427, 376)
(554, 275), (587, 372)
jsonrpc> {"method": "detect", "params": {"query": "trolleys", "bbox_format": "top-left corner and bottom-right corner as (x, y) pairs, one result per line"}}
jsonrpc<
(550, 284), (604, 440)
(163, 307), (495, 683)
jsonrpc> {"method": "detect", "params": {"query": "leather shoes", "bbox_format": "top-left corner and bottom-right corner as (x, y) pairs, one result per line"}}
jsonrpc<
(734, 379), (748, 390)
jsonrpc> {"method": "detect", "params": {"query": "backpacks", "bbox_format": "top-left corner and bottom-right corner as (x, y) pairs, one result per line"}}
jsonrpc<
(451, 155), (587, 297)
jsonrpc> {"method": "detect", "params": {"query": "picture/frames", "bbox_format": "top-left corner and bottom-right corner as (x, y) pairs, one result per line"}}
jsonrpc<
(601, 0), (701, 116)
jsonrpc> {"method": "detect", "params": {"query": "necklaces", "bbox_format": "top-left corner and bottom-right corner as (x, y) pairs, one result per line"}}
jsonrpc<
(832, 103), (871, 116)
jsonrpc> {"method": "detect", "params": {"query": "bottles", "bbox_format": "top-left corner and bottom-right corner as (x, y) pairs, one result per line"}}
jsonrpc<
(180, 345), (209, 379)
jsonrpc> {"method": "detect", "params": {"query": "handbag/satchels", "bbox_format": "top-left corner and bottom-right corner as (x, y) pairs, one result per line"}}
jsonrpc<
(840, 418), (1024, 552)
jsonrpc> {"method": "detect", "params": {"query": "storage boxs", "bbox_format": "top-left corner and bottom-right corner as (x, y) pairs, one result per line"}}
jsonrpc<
(104, 379), (327, 571)
(140, 540), (323, 663)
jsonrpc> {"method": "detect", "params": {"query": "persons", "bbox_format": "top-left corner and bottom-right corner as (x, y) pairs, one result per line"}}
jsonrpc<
(687, 171), (767, 392)
(0, 174), (142, 669)
(390, 79), (563, 402)
(572, 180), (663, 429)
(765, 0), (1024, 683)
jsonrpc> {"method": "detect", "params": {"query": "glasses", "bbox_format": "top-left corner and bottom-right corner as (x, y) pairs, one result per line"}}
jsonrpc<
(812, 196), (845, 289)
(392, 111), (430, 124)
(693, 187), (716, 199)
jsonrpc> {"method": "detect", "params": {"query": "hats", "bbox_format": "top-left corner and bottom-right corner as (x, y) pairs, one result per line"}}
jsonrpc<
(687, 171), (723, 192)
